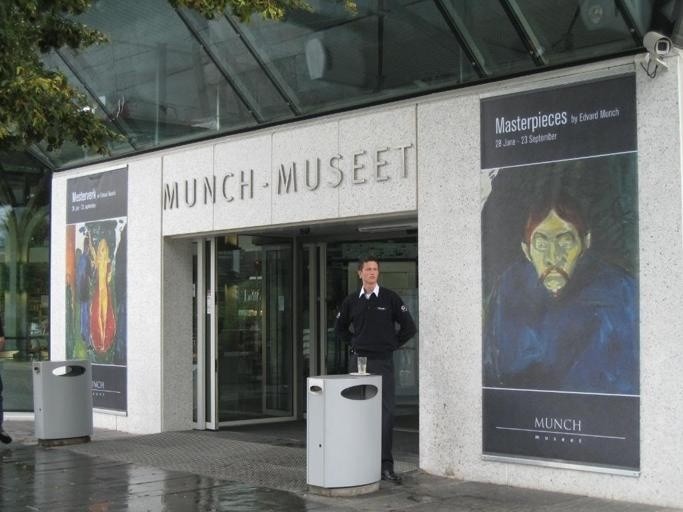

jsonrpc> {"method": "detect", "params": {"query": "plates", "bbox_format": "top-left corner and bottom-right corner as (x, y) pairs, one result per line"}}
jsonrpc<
(349, 372), (370, 376)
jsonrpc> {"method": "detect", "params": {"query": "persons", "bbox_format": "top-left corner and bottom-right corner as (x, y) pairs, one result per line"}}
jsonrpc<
(332, 255), (416, 482)
(481, 193), (635, 396)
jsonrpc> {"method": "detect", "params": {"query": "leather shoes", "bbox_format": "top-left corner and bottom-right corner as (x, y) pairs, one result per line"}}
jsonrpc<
(381, 469), (401, 482)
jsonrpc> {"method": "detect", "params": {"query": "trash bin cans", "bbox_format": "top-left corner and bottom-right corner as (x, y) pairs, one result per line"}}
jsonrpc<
(33, 359), (93, 446)
(306, 375), (383, 498)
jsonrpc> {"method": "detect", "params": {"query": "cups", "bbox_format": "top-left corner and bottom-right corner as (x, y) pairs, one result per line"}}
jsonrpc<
(356, 356), (367, 373)
